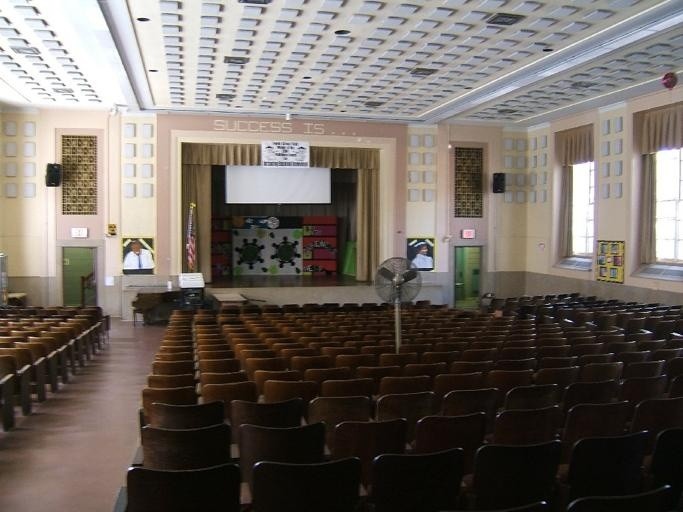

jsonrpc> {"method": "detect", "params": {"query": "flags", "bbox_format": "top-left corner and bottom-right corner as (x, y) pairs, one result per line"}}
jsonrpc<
(186, 203), (197, 271)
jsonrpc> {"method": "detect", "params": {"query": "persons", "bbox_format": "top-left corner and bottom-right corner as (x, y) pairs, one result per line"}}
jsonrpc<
(124, 240), (154, 270)
(410, 242), (433, 269)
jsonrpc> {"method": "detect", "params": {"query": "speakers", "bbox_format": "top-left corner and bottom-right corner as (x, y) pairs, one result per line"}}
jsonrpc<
(492, 173), (505, 193)
(46, 163), (60, 186)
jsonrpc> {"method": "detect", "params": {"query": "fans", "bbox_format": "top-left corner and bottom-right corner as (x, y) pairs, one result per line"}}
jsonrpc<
(375, 257), (422, 354)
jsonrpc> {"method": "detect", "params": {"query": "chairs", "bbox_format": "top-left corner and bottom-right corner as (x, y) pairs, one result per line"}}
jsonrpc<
(0, 306), (109, 432)
(127, 293), (682, 512)
